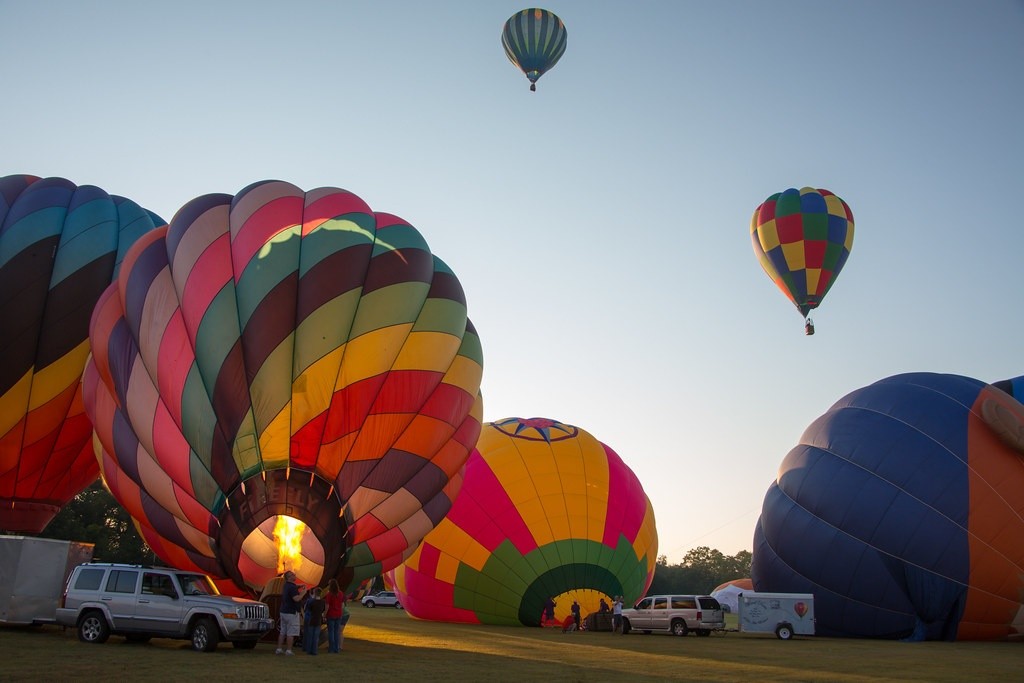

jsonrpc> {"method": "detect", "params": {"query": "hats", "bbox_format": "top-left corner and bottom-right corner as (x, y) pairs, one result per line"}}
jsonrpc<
(574, 601), (577, 603)
(600, 599), (604, 602)
(614, 595), (620, 601)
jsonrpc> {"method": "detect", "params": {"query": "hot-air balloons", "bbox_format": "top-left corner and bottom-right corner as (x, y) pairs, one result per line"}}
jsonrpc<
(750, 186), (856, 335)
(80, 179), (483, 648)
(346, 576), (376, 603)
(751, 371), (1024, 643)
(0, 174), (167, 537)
(390, 417), (659, 633)
(501, 8), (568, 93)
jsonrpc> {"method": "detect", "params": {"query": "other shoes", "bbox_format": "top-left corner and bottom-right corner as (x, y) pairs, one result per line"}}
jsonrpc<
(339, 648), (344, 652)
(275, 649), (286, 655)
(306, 652), (318, 655)
(286, 650), (296, 657)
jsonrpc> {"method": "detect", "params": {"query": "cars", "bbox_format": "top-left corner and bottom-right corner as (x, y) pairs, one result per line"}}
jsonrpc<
(361, 591), (404, 609)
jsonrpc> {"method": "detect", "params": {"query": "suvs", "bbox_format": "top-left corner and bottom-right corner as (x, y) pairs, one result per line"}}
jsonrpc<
(55, 562), (275, 652)
(611, 594), (726, 637)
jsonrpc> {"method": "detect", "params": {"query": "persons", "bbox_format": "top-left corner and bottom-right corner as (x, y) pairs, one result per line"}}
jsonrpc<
(544, 595), (557, 629)
(560, 600), (587, 634)
(274, 571), (306, 656)
(599, 595), (624, 636)
(304, 579), (350, 655)
